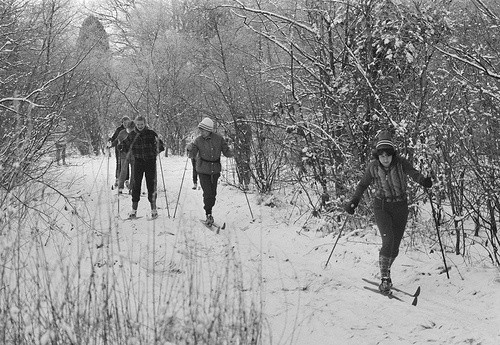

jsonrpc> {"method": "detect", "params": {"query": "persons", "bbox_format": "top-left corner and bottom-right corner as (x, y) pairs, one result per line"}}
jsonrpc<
(186, 117), (233, 225)
(231, 114), (252, 192)
(52, 117), (67, 165)
(186, 140), (201, 191)
(117, 116), (165, 218)
(343, 130), (432, 291)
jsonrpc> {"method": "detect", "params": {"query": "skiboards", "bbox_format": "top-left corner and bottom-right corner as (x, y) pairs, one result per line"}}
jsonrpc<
(361, 278), (420, 306)
(199, 219), (226, 234)
(118, 189), (143, 196)
(56, 164), (78, 167)
(111, 185), (129, 190)
(122, 215), (158, 221)
(192, 184), (203, 190)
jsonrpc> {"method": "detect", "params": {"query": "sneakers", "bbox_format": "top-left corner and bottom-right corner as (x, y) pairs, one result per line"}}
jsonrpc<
(207, 215), (215, 224)
(148, 209), (157, 218)
(381, 277), (395, 295)
(127, 208), (137, 218)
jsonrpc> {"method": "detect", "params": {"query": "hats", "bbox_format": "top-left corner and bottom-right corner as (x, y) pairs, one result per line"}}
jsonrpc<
(197, 117), (214, 132)
(376, 130), (395, 153)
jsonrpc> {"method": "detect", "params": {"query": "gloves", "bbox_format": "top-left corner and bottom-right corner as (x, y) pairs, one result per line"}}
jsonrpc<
(422, 177), (433, 188)
(343, 201), (358, 215)
